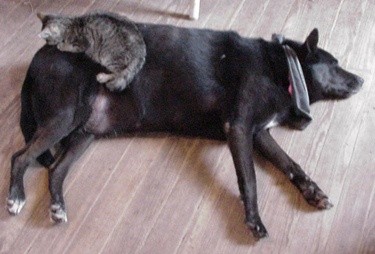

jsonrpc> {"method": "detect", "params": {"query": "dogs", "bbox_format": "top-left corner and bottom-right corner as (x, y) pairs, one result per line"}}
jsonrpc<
(6, 21), (365, 241)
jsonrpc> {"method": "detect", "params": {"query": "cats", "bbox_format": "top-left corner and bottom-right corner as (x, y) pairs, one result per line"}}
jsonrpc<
(35, 8), (146, 95)
